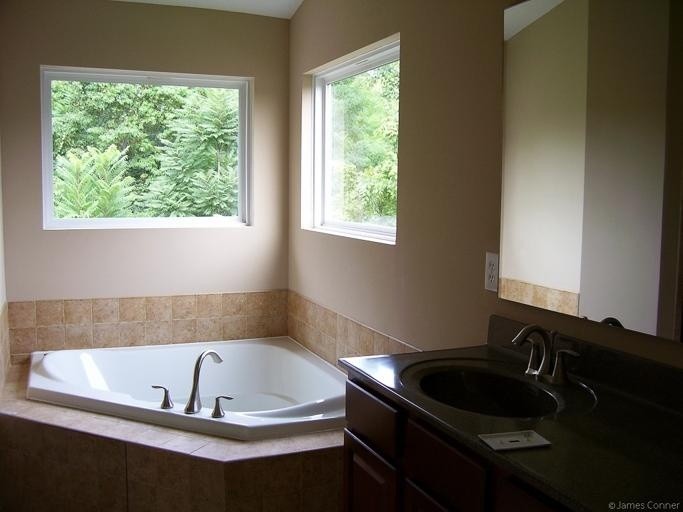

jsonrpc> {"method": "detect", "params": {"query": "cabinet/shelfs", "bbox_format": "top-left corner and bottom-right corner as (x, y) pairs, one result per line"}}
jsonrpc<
(340, 378), (550, 512)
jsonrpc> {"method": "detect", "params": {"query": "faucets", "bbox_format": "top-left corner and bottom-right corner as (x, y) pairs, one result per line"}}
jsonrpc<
(512, 325), (551, 376)
(600, 316), (623, 328)
(184, 350), (224, 413)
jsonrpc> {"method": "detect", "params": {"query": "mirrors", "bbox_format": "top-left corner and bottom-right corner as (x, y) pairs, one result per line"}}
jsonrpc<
(498, 0), (683, 344)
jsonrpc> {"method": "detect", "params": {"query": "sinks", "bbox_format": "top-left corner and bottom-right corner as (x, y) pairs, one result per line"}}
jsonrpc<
(418, 367), (558, 419)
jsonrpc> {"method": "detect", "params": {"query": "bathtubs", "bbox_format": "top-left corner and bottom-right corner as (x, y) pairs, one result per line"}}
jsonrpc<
(26, 335), (347, 440)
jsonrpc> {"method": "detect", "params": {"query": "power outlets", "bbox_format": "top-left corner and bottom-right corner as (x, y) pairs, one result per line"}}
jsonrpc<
(485, 252), (498, 291)
